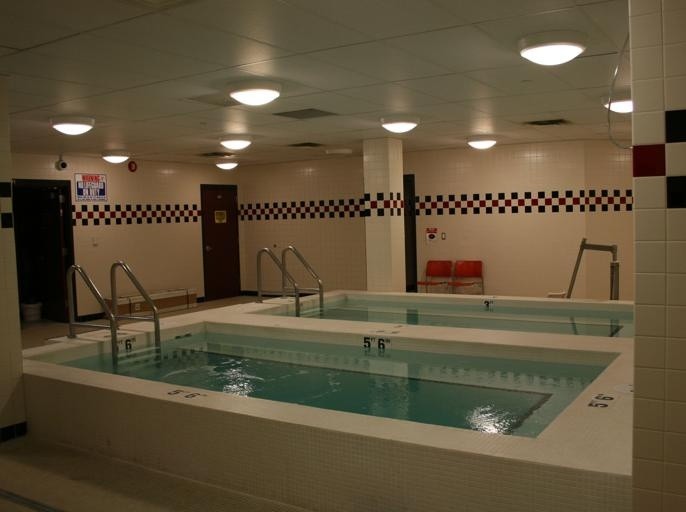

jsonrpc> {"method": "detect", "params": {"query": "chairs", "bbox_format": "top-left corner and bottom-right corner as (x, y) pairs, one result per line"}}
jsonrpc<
(452, 259), (485, 294)
(426, 260), (453, 294)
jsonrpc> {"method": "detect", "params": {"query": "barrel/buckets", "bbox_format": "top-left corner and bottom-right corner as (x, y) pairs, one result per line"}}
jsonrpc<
(21, 302), (42, 322)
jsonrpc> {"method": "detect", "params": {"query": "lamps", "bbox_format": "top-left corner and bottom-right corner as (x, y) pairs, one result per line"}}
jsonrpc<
(519, 28), (587, 67)
(215, 159), (240, 171)
(466, 135), (500, 151)
(225, 79), (282, 107)
(100, 149), (133, 164)
(602, 91), (633, 114)
(379, 114), (420, 135)
(219, 135), (254, 151)
(49, 114), (96, 137)
